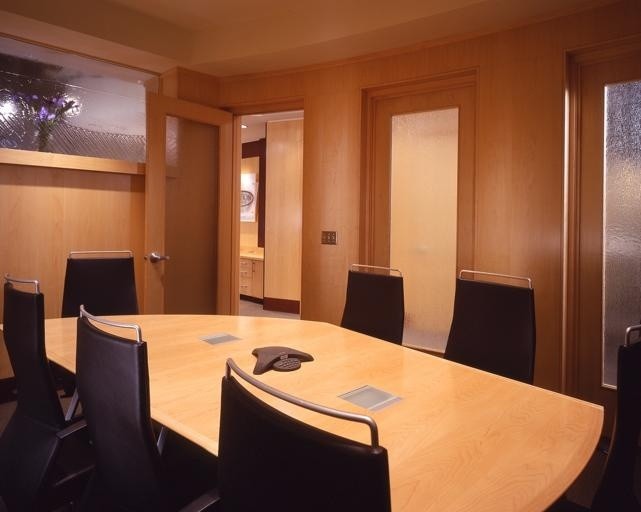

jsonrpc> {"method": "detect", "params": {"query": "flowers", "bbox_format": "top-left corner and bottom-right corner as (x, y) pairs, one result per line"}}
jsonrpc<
(12, 91), (80, 132)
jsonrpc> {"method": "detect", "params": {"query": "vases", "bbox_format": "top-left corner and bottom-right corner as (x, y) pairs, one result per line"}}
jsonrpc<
(37, 130), (54, 151)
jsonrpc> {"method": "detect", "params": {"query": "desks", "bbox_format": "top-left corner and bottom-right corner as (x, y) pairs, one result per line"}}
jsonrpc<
(50, 311), (607, 512)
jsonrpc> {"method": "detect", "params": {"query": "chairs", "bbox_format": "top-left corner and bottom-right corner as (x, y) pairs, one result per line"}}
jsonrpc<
(60, 249), (144, 421)
(36, 304), (168, 510)
(191, 358), (391, 512)
(1, 272), (66, 492)
(441, 268), (538, 385)
(341, 263), (406, 346)
(591, 323), (641, 511)
